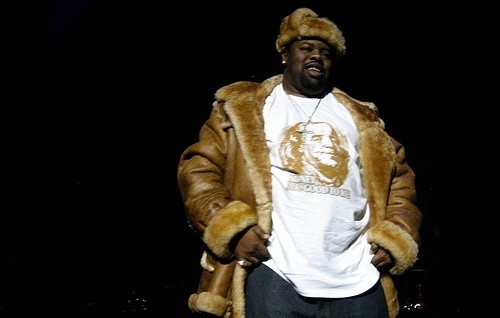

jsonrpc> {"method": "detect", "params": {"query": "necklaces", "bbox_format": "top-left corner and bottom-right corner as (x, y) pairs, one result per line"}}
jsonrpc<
(284, 86), (327, 133)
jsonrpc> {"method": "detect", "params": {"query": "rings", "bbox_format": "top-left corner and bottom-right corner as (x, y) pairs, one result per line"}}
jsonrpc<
(237, 259), (246, 265)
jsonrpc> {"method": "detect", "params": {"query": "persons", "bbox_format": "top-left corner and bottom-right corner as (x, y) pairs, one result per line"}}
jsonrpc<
(177, 8), (422, 318)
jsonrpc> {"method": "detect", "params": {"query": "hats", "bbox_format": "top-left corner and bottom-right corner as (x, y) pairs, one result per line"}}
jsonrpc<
(275, 6), (349, 63)
